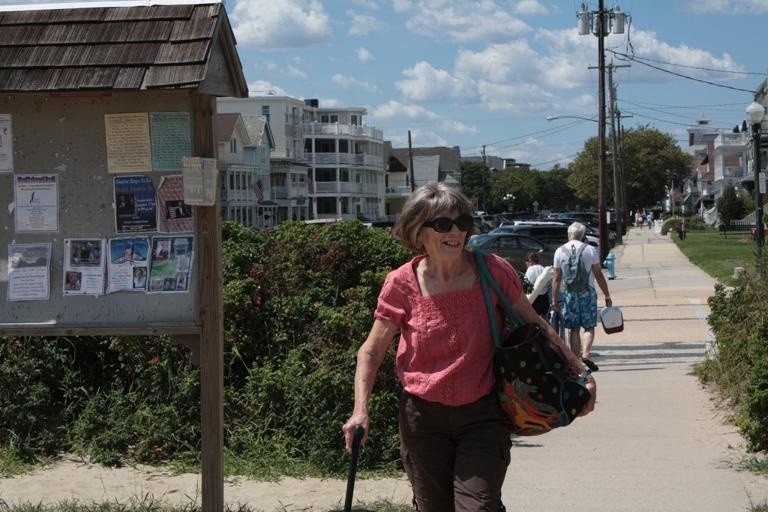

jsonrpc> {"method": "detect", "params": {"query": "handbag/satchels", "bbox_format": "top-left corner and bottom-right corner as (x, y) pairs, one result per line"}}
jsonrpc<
(473, 249), (592, 436)
(599, 304), (623, 334)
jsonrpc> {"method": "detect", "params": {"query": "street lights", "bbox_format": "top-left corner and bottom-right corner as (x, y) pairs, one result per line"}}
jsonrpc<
(547, 116), (625, 244)
(746, 103), (765, 276)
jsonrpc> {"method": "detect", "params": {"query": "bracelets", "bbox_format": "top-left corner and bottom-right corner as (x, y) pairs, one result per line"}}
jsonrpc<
(581, 367), (592, 379)
(605, 296), (611, 300)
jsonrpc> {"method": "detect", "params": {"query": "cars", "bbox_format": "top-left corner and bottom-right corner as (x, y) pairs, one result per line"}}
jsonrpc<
(467, 208), (625, 273)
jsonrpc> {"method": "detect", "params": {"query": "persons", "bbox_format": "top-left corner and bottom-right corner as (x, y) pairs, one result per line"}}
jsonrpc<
(132, 267), (146, 288)
(522, 251), (550, 318)
(634, 209), (640, 228)
(342, 180), (597, 512)
(551, 220), (624, 370)
(638, 214), (644, 230)
(174, 200), (188, 219)
(646, 214), (653, 229)
(66, 272), (80, 292)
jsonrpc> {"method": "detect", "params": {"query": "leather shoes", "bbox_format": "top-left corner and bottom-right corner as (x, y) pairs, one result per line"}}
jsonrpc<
(583, 359), (598, 372)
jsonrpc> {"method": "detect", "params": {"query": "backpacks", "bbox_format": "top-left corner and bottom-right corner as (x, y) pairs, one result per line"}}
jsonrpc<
(560, 242), (591, 292)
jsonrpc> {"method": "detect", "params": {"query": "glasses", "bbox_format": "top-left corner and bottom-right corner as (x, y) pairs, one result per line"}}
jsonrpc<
(421, 214), (475, 235)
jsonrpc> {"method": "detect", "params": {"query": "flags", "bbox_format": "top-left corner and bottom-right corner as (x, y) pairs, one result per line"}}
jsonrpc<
(253, 180), (264, 205)
